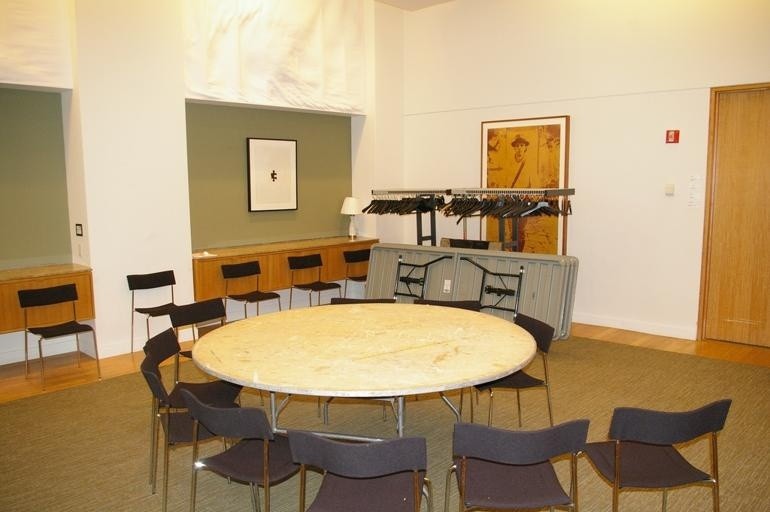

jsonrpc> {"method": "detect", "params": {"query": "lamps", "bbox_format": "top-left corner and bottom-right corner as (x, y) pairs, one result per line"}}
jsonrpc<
(340, 195), (360, 239)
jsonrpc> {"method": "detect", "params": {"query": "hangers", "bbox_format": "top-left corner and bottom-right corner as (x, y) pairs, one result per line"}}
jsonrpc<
(439, 190), (574, 225)
(360, 189), (449, 217)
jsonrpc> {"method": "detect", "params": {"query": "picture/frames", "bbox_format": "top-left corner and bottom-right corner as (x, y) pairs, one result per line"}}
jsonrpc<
(477, 114), (571, 256)
(245, 136), (300, 213)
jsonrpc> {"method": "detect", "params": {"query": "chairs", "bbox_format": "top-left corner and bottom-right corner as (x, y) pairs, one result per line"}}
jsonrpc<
(443, 417), (592, 512)
(15, 282), (103, 386)
(177, 385), (304, 510)
(583, 398), (732, 511)
(474, 312), (556, 411)
(286, 253), (342, 308)
(137, 351), (245, 512)
(126, 269), (175, 355)
(142, 326), (244, 487)
(219, 260), (282, 320)
(285, 427), (436, 512)
(168, 296), (227, 384)
(343, 248), (371, 297)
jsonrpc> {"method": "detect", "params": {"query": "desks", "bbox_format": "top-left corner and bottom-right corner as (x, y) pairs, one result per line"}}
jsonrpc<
(192, 301), (538, 445)
